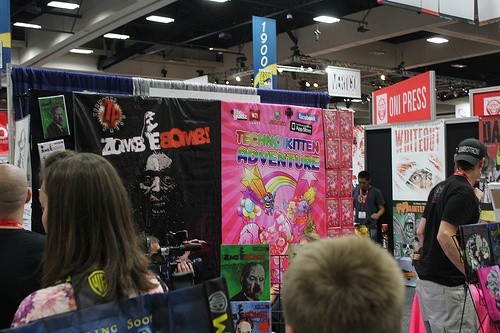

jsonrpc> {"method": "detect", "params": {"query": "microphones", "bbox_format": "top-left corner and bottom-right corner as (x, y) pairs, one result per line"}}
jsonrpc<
(179, 244), (202, 250)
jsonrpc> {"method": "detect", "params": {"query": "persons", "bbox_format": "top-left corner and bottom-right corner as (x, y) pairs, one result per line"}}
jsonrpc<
(413, 138), (487, 333)
(42, 149), (75, 178)
(230, 262), (266, 301)
(352, 170), (386, 246)
(10, 151), (168, 330)
(46, 106), (65, 137)
(0, 163), (46, 329)
(281, 234), (404, 333)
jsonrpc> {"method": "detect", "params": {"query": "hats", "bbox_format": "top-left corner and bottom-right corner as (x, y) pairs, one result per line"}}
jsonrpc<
(454, 138), (486, 165)
